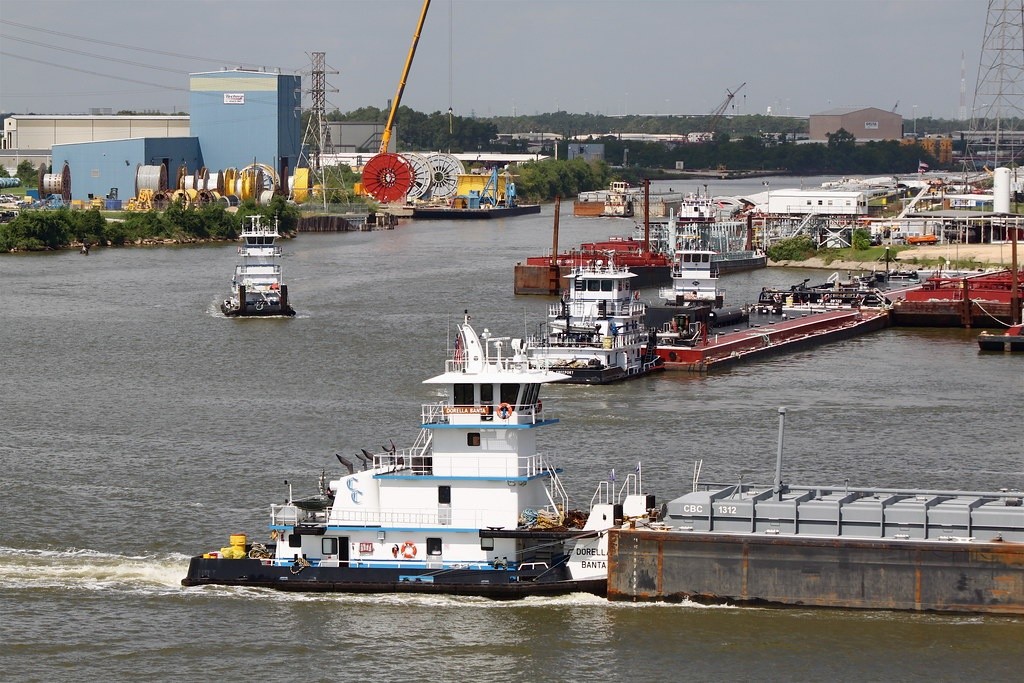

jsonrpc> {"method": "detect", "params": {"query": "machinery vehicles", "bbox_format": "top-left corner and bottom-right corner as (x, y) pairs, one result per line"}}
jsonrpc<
(681, 82), (746, 143)
(353, 0), (453, 199)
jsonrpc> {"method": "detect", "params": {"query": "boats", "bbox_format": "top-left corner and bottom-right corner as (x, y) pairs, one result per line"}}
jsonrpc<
(180, 303), (664, 604)
(218, 214), (297, 317)
(523, 174), (920, 385)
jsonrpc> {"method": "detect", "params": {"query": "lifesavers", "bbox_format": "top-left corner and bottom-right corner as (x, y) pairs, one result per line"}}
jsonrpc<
(401, 541), (417, 559)
(497, 403), (512, 419)
